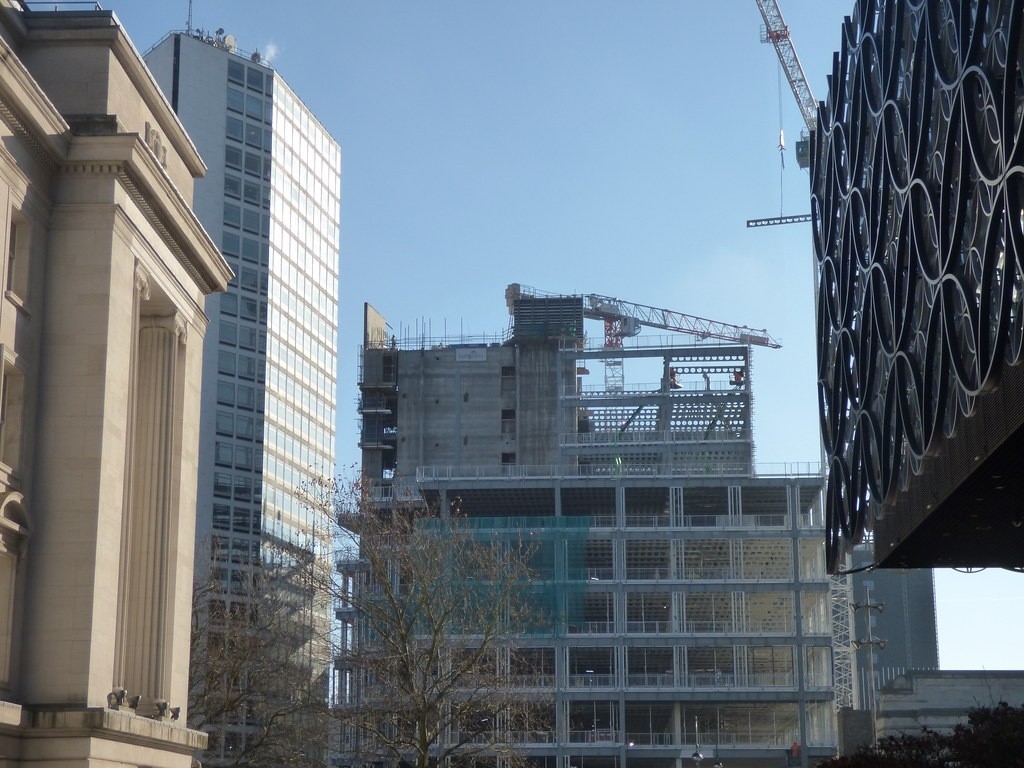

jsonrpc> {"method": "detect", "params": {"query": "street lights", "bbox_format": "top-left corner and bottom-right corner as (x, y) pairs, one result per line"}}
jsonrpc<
(692, 746), (704, 768)
(849, 602), (889, 753)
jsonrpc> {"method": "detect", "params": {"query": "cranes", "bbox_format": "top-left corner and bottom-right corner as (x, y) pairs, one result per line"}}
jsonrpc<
(747, 0), (823, 234)
(504, 282), (784, 394)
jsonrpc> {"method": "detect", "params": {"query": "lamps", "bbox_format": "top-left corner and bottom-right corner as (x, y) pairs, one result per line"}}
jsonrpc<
(107, 689), (180, 722)
